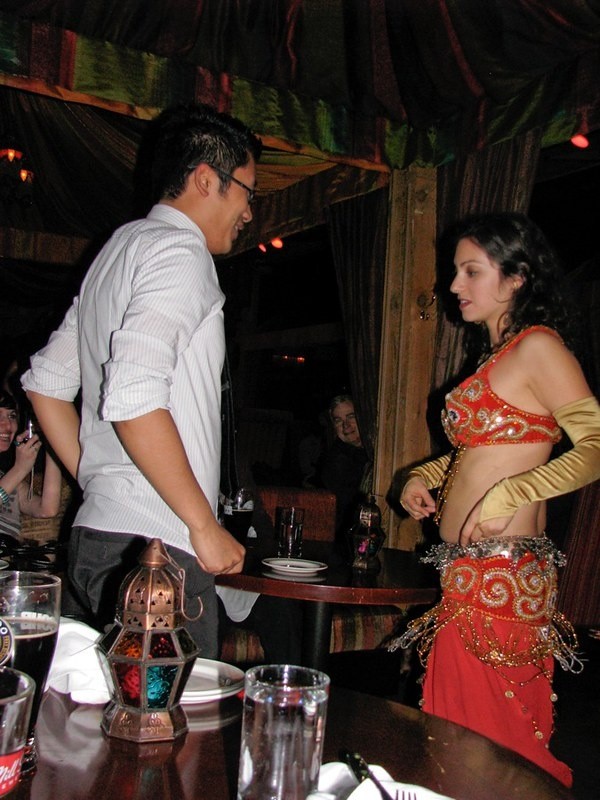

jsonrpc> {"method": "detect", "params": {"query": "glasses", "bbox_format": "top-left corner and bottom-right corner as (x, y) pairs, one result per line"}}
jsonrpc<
(184, 162), (257, 204)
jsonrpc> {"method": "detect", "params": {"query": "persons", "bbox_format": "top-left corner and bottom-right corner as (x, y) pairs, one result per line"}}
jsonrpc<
(399, 210), (600, 788)
(1, 392), (365, 666)
(20, 104), (262, 659)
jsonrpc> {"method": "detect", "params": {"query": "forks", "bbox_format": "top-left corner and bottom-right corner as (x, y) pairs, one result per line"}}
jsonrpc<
(395, 789), (417, 800)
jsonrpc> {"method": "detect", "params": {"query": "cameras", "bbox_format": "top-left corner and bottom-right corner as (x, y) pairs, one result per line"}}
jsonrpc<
(25, 420), (36, 441)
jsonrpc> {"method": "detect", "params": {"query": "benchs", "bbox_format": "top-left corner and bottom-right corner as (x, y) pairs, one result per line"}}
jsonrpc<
(221, 488), (416, 669)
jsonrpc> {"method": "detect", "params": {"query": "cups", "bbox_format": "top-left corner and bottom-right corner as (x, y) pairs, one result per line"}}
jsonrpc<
(273, 506), (305, 558)
(236, 665), (330, 800)
(0, 570), (62, 781)
(0, 666), (36, 798)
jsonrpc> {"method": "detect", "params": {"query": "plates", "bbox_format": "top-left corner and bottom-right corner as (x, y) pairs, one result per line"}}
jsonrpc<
(0, 560), (10, 570)
(261, 558), (328, 573)
(180, 657), (245, 704)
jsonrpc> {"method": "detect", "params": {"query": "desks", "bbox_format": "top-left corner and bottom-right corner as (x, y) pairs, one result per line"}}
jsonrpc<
(0, 686), (577, 800)
(214, 539), (442, 675)
